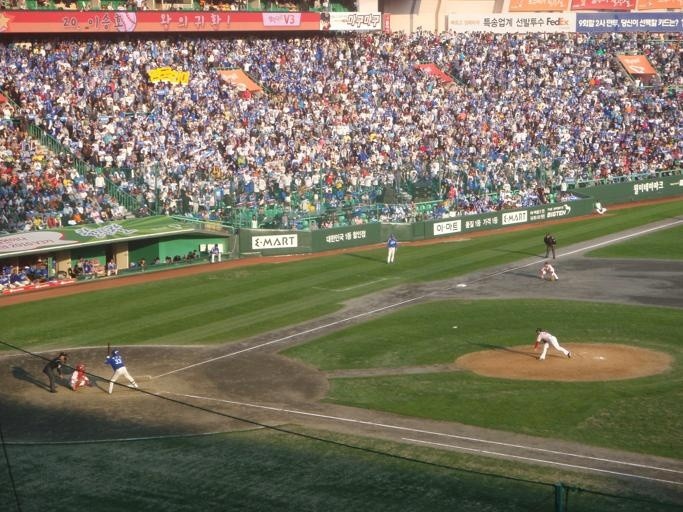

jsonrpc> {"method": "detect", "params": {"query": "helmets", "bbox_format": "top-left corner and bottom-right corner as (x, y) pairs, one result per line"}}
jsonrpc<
(76, 363), (85, 371)
(59, 352), (68, 363)
(112, 349), (120, 357)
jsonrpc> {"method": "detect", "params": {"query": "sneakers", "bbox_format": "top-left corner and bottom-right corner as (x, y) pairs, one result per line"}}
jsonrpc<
(567, 352), (570, 359)
(50, 390), (58, 393)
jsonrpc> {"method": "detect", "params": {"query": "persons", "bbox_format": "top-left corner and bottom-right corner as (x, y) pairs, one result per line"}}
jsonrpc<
(0, 244), (221, 291)
(1, 0), (680, 216)
(386, 233), (396, 263)
(103, 349), (138, 394)
(543, 232), (556, 259)
(42, 350), (66, 392)
(533, 328), (571, 360)
(67, 363), (90, 391)
(536, 261), (559, 280)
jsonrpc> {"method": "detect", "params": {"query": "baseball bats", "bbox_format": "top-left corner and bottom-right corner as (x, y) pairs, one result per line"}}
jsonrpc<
(108, 342), (110, 357)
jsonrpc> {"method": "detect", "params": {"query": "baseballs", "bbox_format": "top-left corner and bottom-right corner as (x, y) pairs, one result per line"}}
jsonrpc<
(452, 326), (458, 329)
